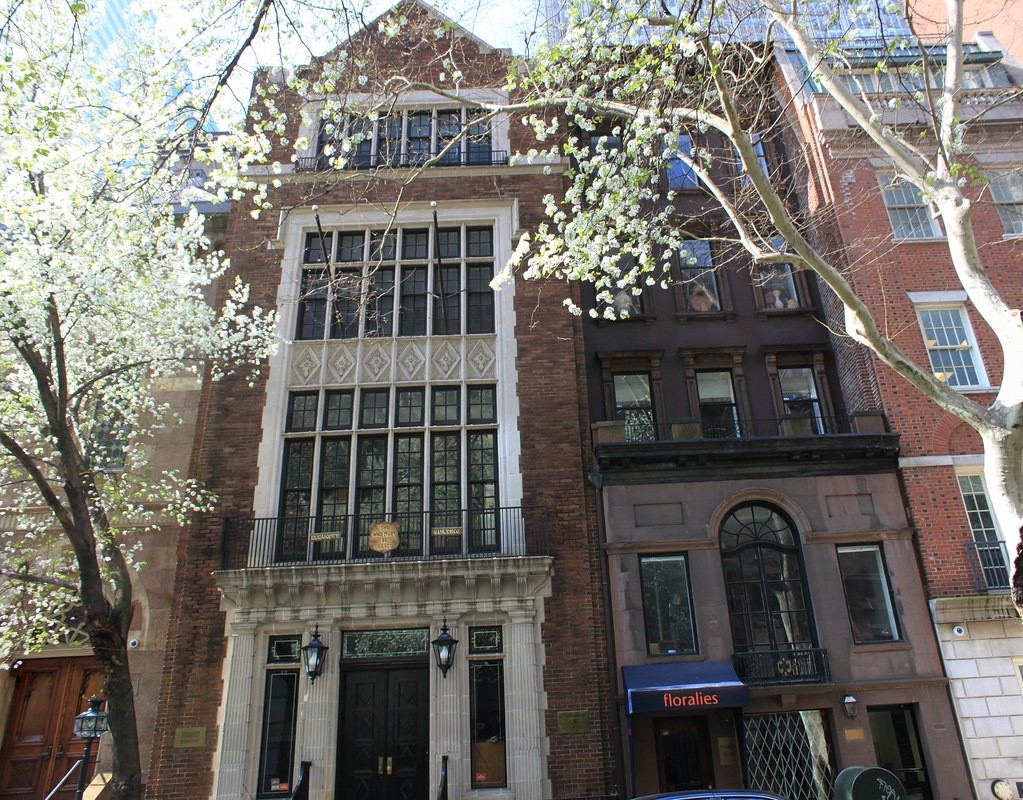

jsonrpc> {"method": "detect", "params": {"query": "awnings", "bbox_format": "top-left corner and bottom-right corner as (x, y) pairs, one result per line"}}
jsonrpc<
(622, 661), (750, 714)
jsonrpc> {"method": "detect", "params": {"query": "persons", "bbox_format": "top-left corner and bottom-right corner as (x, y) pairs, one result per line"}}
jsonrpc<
(1011, 526), (1023, 613)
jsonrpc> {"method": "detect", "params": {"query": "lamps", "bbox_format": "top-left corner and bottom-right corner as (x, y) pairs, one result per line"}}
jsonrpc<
(431, 616), (459, 679)
(841, 695), (857, 720)
(302, 622), (329, 685)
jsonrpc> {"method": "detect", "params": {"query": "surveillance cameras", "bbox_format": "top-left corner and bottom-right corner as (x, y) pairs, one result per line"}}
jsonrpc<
(952, 626), (965, 637)
(130, 639), (139, 649)
